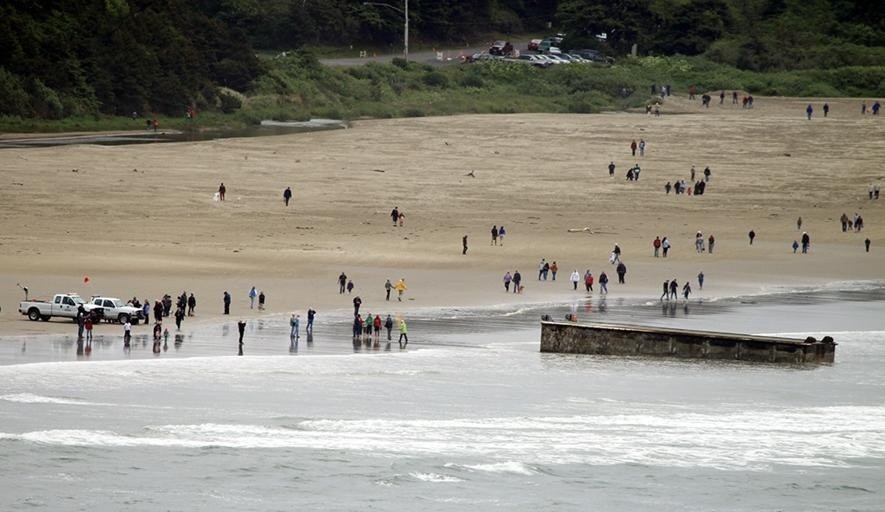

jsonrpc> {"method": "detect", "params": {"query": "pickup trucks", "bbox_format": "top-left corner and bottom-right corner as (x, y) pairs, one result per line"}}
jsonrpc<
(84, 294), (144, 326)
(16, 292), (107, 325)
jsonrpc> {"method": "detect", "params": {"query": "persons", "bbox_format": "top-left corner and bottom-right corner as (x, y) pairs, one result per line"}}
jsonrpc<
(284, 187), (292, 206)
(645, 83), (753, 117)
(807, 103), (829, 120)
(862, 101), (881, 114)
(607, 139), (711, 196)
(219, 183), (226, 201)
(186, 109), (194, 119)
(792, 182), (880, 253)
(503, 231), (715, 299)
(462, 235), (468, 255)
(78, 272), (409, 345)
(146, 119), (158, 132)
(490, 225), (506, 247)
(391, 206), (405, 227)
(749, 230), (755, 245)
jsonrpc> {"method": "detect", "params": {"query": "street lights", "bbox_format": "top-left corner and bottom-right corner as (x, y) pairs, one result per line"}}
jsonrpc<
(363, 0), (410, 63)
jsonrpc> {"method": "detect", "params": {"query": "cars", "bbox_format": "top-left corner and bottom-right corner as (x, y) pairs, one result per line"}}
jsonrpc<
(517, 31), (619, 69)
(488, 38), (514, 56)
